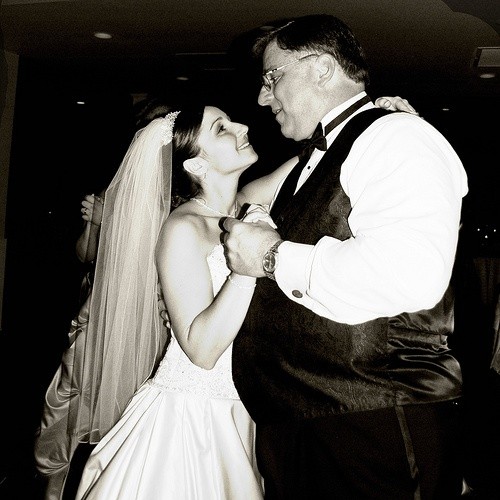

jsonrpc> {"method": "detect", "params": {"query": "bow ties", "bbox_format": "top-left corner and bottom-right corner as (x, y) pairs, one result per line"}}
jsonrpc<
(294, 95), (371, 163)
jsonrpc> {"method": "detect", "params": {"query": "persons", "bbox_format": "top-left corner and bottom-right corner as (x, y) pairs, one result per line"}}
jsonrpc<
(34, 95), (421, 500)
(158, 15), (476, 500)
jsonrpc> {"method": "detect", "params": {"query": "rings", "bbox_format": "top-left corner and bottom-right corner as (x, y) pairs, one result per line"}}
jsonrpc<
(83, 208), (88, 214)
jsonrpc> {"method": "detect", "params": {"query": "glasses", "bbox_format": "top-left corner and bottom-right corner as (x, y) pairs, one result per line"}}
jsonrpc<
(261, 53), (320, 92)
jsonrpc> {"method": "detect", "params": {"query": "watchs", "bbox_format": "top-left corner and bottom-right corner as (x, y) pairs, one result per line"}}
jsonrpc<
(261, 238), (286, 281)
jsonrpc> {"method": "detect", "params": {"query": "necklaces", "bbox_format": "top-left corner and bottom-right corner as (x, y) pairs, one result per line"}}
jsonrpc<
(192, 197), (237, 219)
